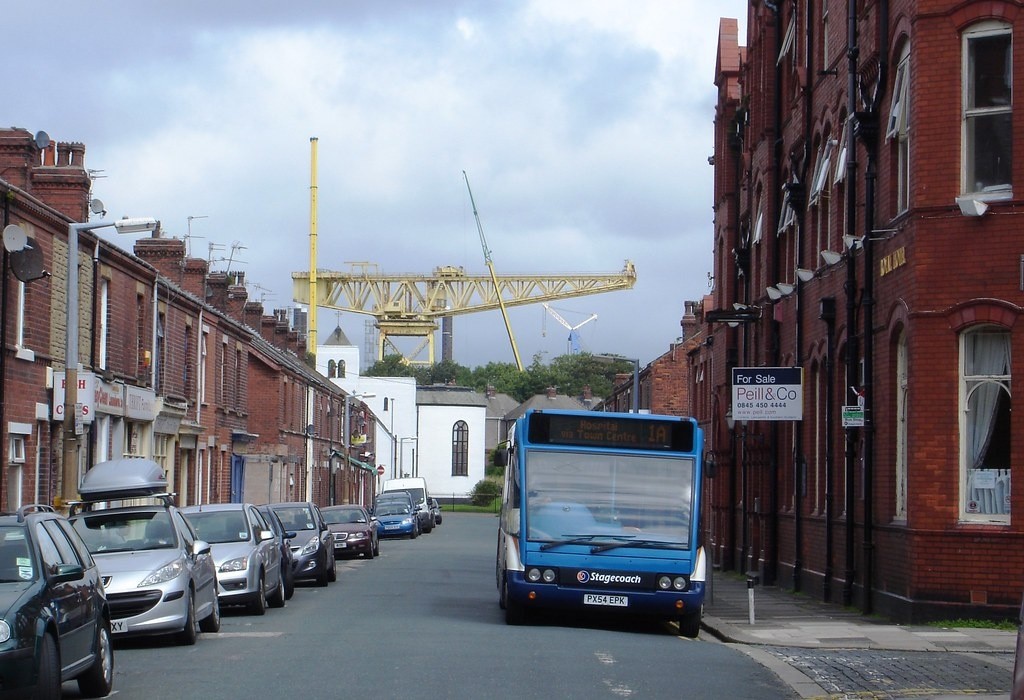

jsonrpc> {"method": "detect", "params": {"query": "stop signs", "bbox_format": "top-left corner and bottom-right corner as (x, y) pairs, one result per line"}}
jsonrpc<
(376, 466), (384, 475)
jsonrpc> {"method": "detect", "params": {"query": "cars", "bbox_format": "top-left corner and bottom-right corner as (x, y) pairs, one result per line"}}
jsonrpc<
(316, 503), (381, 560)
(428, 498), (443, 525)
(263, 501), (335, 589)
(251, 505), (298, 603)
(170, 502), (287, 617)
(60, 458), (221, 647)
(368, 500), (419, 540)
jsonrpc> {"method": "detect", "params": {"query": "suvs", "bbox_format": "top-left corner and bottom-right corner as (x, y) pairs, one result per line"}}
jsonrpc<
(1, 503), (117, 700)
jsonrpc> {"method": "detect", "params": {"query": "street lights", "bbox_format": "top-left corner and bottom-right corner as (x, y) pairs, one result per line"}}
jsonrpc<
(49, 217), (159, 514)
(336, 392), (376, 505)
(399, 436), (419, 479)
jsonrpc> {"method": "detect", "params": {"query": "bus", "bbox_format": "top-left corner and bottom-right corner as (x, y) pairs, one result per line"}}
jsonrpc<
(489, 408), (718, 639)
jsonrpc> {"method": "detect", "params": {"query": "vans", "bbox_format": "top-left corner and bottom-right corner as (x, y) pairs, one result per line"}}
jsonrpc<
(371, 490), (422, 538)
(376, 477), (433, 535)
